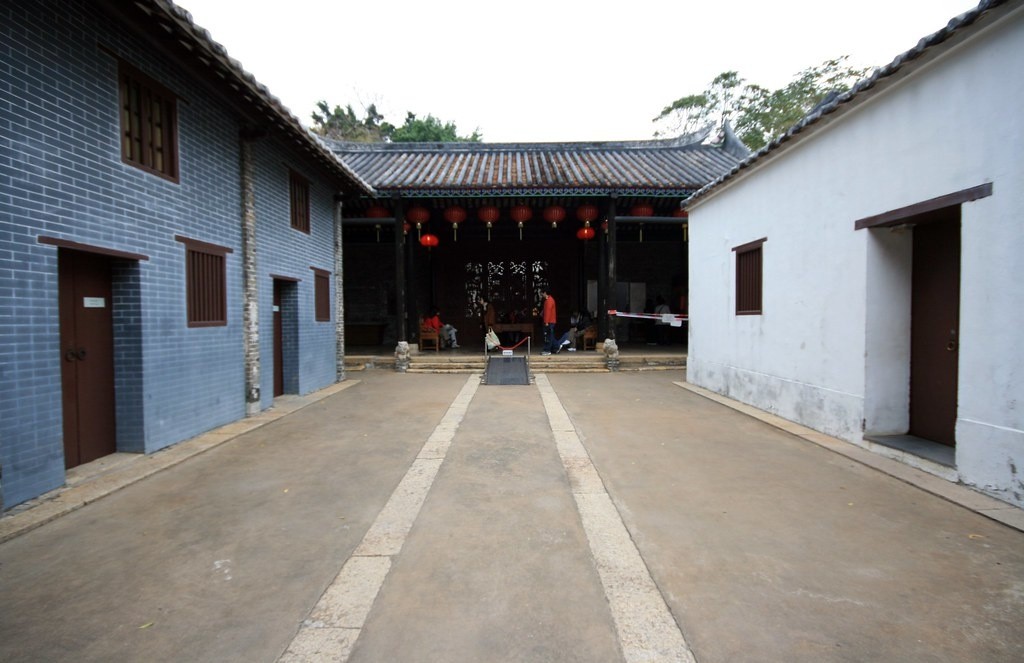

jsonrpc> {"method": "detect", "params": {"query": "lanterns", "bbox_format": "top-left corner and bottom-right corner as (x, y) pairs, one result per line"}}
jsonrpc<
(365, 207), (391, 243)
(406, 205), (430, 241)
(543, 205), (566, 229)
(673, 208), (688, 240)
(510, 204), (533, 241)
(577, 228), (595, 240)
(419, 233), (439, 251)
(631, 203), (653, 243)
(444, 205), (467, 242)
(601, 221), (608, 243)
(478, 205), (499, 242)
(576, 205), (599, 227)
(403, 221), (410, 244)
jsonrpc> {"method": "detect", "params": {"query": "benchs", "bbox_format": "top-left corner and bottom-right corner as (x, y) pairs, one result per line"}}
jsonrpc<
(418, 315), (439, 351)
(581, 320), (597, 350)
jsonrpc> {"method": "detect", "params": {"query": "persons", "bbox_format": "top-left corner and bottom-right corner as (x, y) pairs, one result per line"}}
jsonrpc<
(558, 309), (593, 352)
(432, 311), (461, 349)
(653, 295), (673, 345)
(539, 285), (563, 355)
(477, 296), (500, 352)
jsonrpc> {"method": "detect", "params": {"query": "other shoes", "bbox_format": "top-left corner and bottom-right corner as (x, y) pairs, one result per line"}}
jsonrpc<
(555, 344), (563, 354)
(567, 348), (577, 352)
(451, 344), (460, 348)
(541, 351), (551, 355)
(452, 328), (458, 332)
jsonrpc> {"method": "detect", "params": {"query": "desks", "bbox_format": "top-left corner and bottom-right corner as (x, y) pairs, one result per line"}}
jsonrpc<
(493, 323), (534, 346)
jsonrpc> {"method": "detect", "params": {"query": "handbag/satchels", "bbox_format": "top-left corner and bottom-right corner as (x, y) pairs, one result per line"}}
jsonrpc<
(485, 328), (500, 349)
